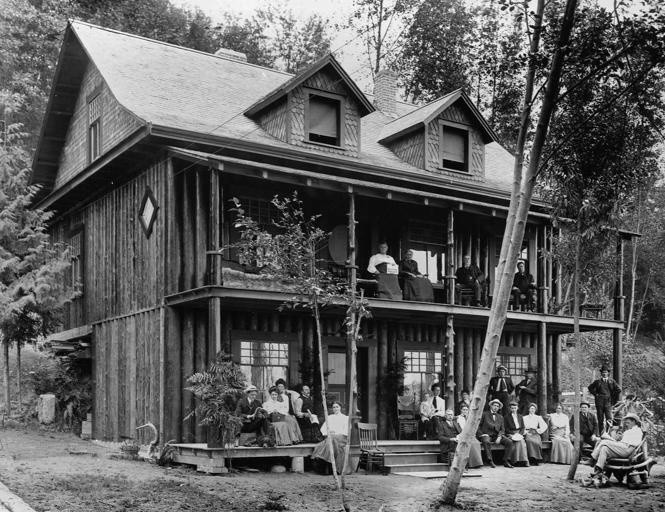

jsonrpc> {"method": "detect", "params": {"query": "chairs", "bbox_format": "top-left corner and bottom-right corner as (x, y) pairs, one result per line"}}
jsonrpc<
(599, 431), (656, 484)
(396, 392), (420, 441)
(329, 261), (534, 314)
(353, 421), (386, 476)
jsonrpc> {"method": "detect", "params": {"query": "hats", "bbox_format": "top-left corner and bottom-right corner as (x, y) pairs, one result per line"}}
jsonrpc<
(496, 364), (508, 371)
(623, 413), (639, 423)
(528, 402), (538, 408)
(488, 398), (504, 410)
(580, 401), (590, 408)
(599, 365), (611, 372)
(509, 401), (518, 405)
(525, 366), (538, 373)
(243, 385), (259, 395)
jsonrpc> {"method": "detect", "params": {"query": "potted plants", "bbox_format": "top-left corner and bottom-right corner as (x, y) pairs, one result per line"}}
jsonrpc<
(184, 352), (256, 449)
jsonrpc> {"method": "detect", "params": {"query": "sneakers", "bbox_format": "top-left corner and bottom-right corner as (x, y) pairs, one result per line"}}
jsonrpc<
(475, 300), (483, 307)
(580, 458), (595, 467)
(490, 461), (496, 468)
(514, 306), (534, 313)
(504, 462), (514, 468)
(589, 466), (602, 476)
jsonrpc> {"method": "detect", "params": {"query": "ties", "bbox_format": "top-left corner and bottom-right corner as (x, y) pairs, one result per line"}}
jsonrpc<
(432, 396), (438, 412)
(583, 412), (586, 417)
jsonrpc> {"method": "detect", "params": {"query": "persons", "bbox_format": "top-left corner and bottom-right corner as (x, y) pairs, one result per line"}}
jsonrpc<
(366, 240), (403, 301)
(513, 262), (537, 312)
(489, 365), (515, 407)
(455, 253), (489, 309)
(398, 249), (435, 303)
(580, 412), (644, 478)
(235, 376), (324, 441)
(312, 400), (353, 474)
(515, 365), (538, 434)
(420, 382), (598, 468)
(589, 366), (623, 438)
